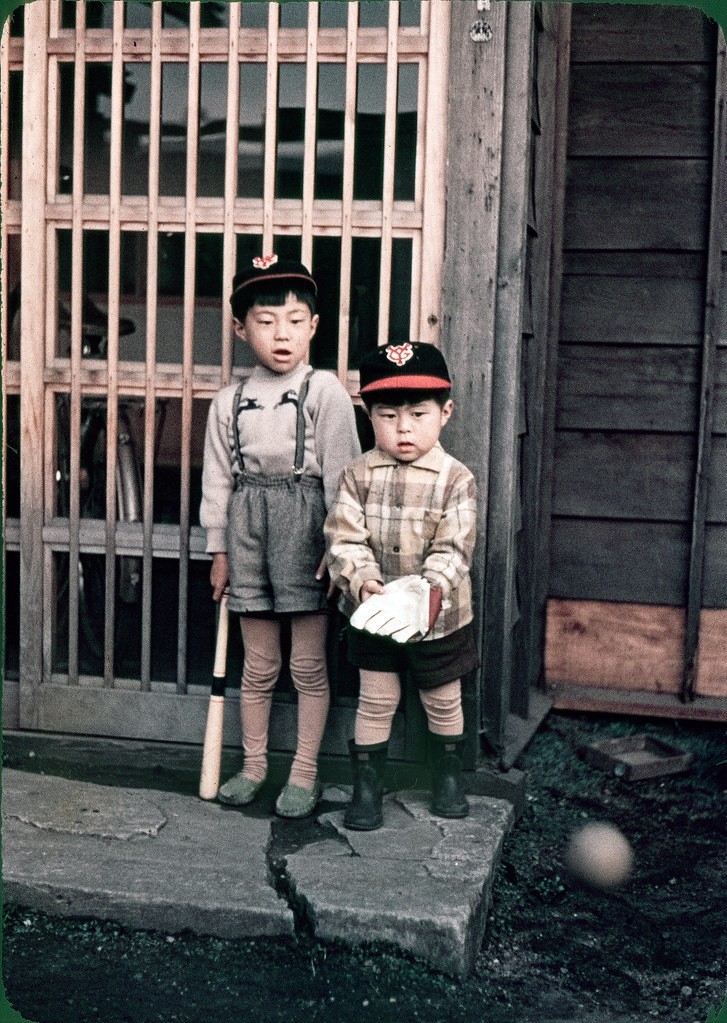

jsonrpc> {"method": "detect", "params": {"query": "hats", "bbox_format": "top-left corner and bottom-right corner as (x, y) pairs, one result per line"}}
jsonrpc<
(358, 340), (452, 392)
(230, 254), (317, 304)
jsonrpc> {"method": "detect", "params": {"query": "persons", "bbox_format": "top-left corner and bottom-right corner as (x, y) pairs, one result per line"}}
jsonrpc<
(198, 254), (362, 818)
(322, 342), (477, 831)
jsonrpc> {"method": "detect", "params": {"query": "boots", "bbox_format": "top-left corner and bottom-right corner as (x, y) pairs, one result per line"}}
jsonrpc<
(344, 739), (384, 830)
(432, 734), (470, 818)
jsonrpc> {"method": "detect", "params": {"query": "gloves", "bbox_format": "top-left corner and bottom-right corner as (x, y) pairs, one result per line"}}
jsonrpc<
(350, 574), (443, 644)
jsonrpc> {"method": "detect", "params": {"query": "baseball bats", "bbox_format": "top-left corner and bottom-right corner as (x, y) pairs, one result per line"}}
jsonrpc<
(201, 591), (231, 798)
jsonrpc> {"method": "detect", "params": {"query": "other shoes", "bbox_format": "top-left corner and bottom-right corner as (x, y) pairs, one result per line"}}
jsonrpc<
(276, 774), (320, 818)
(217, 771), (271, 806)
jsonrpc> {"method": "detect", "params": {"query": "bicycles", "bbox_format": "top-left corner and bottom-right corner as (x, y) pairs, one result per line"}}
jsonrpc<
(3, 167), (174, 674)
